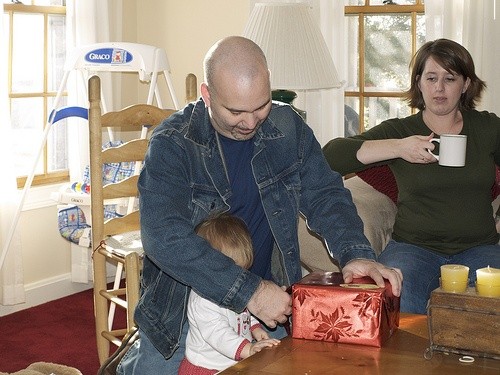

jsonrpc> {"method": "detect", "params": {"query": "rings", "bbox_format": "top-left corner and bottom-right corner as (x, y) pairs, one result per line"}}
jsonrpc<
(422, 156), (424, 160)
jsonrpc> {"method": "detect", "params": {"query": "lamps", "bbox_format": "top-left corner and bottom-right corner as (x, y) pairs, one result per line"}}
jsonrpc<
(239, 1), (344, 123)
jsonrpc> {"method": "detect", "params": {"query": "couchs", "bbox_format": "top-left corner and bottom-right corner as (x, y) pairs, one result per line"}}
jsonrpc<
(295, 165), (500, 273)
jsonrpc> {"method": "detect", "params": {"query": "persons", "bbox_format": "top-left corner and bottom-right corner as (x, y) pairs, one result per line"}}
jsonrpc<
(321, 39), (500, 313)
(91, 36), (404, 375)
(179, 215), (281, 375)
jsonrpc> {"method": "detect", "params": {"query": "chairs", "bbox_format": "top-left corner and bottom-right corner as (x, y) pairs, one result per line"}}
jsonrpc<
(88, 73), (198, 368)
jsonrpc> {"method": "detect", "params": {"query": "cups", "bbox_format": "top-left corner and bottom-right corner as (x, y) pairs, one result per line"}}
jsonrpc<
(474, 280), (500, 300)
(427, 132), (467, 167)
(439, 277), (471, 294)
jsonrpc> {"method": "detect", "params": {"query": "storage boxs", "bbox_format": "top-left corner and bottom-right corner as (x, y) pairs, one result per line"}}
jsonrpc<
(290, 273), (400, 349)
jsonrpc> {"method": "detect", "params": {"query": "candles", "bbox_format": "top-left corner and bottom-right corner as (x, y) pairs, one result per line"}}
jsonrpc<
(440, 264), (470, 293)
(476, 265), (500, 299)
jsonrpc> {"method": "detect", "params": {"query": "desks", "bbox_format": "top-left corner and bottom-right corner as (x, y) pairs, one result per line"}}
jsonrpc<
(214, 312), (500, 375)
